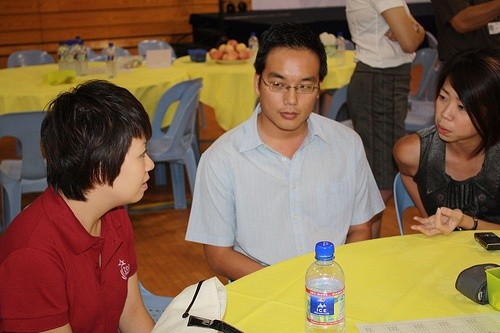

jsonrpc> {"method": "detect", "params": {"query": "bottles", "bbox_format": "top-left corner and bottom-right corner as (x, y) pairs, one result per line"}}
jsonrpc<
(56, 34), (88, 75)
(104, 41), (115, 79)
(304, 240), (346, 333)
(337, 33), (346, 51)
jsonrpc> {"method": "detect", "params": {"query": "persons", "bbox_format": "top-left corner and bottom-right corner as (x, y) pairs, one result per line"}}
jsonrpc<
(392, 51), (500, 236)
(185, 23), (386, 279)
(344, 0), (500, 206)
(0, 79), (156, 333)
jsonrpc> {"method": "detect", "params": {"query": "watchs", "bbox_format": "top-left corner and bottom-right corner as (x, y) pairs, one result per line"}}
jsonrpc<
(457, 214), (478, 230)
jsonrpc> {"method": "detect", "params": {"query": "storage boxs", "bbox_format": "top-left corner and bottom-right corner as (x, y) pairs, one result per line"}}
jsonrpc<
(484, 267), (500, 311)
(475, 232), (500, 250)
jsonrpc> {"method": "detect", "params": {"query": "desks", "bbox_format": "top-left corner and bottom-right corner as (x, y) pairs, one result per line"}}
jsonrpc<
(219, 231), (500, 333)
(0, 55), (191, 132)
(180, 50), (357, 132)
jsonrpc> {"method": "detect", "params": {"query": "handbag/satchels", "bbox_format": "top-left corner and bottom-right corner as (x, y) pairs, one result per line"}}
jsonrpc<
(150, 277), (243, 333)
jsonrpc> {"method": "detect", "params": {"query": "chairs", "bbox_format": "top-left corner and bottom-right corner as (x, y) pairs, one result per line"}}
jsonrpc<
(0, 31), (438, 326)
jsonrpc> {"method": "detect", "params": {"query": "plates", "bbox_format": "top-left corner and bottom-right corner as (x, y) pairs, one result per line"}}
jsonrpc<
(215, 58), (250, 65)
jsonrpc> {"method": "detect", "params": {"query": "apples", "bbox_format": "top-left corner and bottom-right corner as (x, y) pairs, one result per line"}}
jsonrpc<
(209, 39), (251, 60)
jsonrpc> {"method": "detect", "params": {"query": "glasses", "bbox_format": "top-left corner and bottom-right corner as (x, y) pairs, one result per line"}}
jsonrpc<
(261, 76), (320, 94)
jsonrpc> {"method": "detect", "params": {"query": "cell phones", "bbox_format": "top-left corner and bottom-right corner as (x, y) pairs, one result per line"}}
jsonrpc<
(475, 232), (500, 250)
